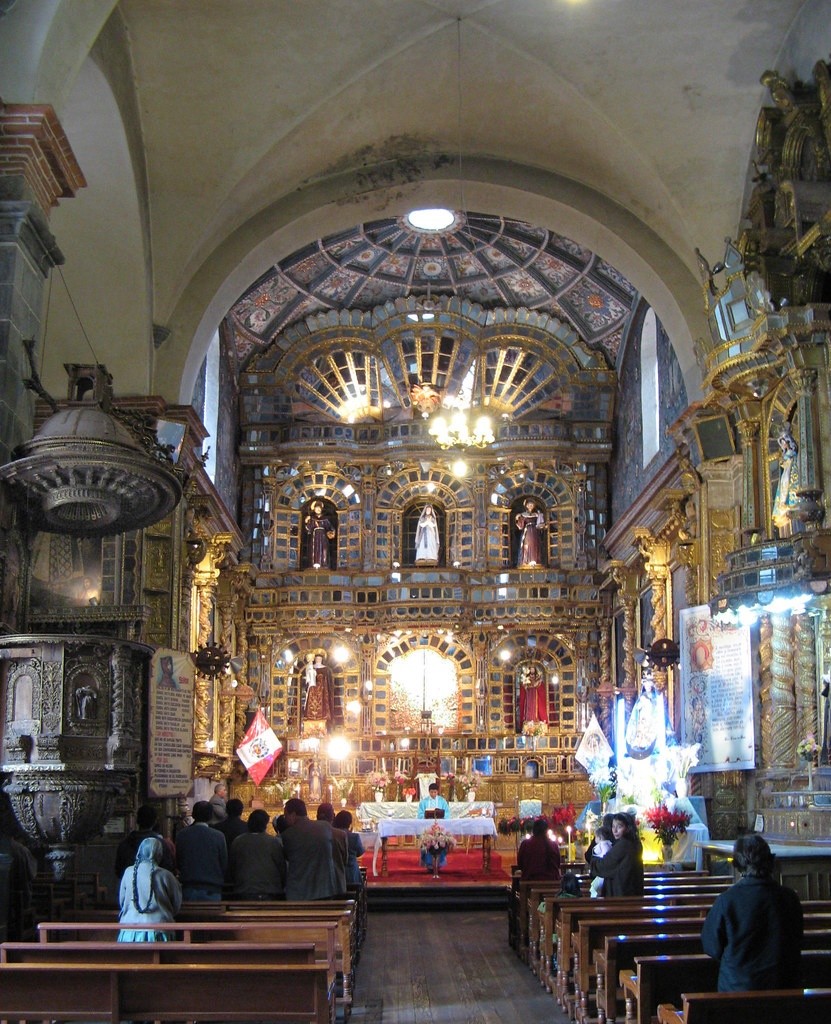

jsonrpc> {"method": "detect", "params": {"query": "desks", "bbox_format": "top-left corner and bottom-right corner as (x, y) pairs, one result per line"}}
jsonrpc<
(347, 828), (382, 877)
(355, 799), (497, 855)
(371, 817), (496, 877)
(663, 795), (708, 825)
(643, 823), (711, 870)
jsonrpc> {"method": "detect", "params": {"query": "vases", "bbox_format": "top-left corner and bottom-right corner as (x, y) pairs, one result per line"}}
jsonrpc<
(342, 797), (347, 807)
(602, 800), (608, 814)
(406, 793), (413, 802)
(624, 802), (637, 817)
(674, 779), (687, 798)
(809, 762), (814, 791)
(528, 735), (538, 751)
(565, 842), (576, 860)
(453, 792), (458, 801)
(661, 842), (674, 863)
(467, 790), (475, 801)
(432, 847), (440, 878)
(580, 844), (589, 860)
(656, 797), (661, 808)
(375, 790), (384, 801)
(283, 797), (289, 808)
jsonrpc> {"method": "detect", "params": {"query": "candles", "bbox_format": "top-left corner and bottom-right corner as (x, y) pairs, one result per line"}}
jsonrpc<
(587, 822), (591, 845)
(565, 826), (572, 860)
(330, 785), (332, 793)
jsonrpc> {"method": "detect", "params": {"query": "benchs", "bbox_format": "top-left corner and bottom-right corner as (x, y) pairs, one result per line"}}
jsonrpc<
(509, 861), (831, 1024)
(0, 865), (369, 1024)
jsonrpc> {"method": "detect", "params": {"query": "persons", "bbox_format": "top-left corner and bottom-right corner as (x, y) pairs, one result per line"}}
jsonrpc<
(520, 662), (548, 729)
(415, 505), (439, 560)
(272, 798), (365, 900)
(303, 648), (335, 721)
(175, 801), (228, 902)
(537, 873), (583, 976)
(417, 783), (450, 870)
(0, 810), (35, 929)
(517, 819), (561, 881)
(75, 685), (97, 719)
(115, 805), (176, 901)
(626, 672), (666, 786)
(585, 812), (644, 898)
(772, 433), (798, 538)
(701, 836), (804, 992)
(515, 497), (545, 565)
(213, 799), (249, 844)
(304, 500), (336, 569)
(228, 809), (287, 902)
(117, 837), (182, 942)
(207, 784), (227, 824)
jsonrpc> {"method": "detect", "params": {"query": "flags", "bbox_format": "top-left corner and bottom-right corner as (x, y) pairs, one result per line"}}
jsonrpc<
(575, 712), (614, 775)
(236, 710), (282, 786)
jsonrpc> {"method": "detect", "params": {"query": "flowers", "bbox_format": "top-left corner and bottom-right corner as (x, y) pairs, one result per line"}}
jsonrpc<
(664, 743), (701, 778)
(618, 787), (640, 805)
(520, 720), (550, 737)
(395, 770), (416, 794)
(499, 806), (577, 840)
(364, 769), (391, 791)
(648, 806), (693, 845)
(334, 779), (354, 798)
(577, 828), (596, 846)
(590, 768), (616, 802)
(418, 821), (456, 853)
(650, 785), (668, 801)
(446, 774), (457, 796)
(459, 770), (483, 792)
(796, 734), (821, 763)
(266, 778), (301, 800)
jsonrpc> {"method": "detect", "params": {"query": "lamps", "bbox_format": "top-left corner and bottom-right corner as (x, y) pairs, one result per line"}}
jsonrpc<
(89, 597), (98, 605)
(427, 271), (498, 455)
